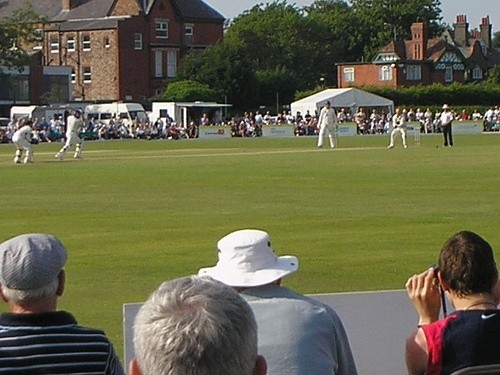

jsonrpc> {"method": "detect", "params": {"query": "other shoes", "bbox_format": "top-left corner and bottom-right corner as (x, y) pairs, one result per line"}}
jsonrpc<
(55, 155), (64, 161)
(404, 144), (408, 149)
(388, 145), (394, 148)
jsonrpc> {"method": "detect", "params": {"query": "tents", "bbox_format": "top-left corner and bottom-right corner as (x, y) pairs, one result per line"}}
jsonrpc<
(291, 87), (394, 120)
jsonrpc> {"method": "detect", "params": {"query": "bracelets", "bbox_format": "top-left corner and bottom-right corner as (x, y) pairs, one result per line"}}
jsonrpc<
(417, 324), (425, 329)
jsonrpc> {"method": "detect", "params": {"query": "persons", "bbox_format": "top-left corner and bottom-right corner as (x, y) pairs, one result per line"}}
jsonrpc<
(55, 110), (83, 160)
(387, 109), (408, 150)
(198, 229), (358, 375)
(405, 231), (500, 375)
(0, 106), (209, 144)
(0, 233), (125, 375)
(436, 104), (453, 147)
(316, 101), (338, 148)
(12, 120), (34, 163)
(129, 275), (267, 375)
(223, 107), (500, 137)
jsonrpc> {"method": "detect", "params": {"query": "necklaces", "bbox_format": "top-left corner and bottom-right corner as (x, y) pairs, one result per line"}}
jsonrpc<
(465, 301), (497, 309)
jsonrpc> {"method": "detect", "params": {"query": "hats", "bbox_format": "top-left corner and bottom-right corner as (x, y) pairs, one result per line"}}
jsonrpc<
(0, 233), (68, 291)
(199, 228), (299, 288)
(442, 103), (449, 108)
(325, 101), (330, 105)
